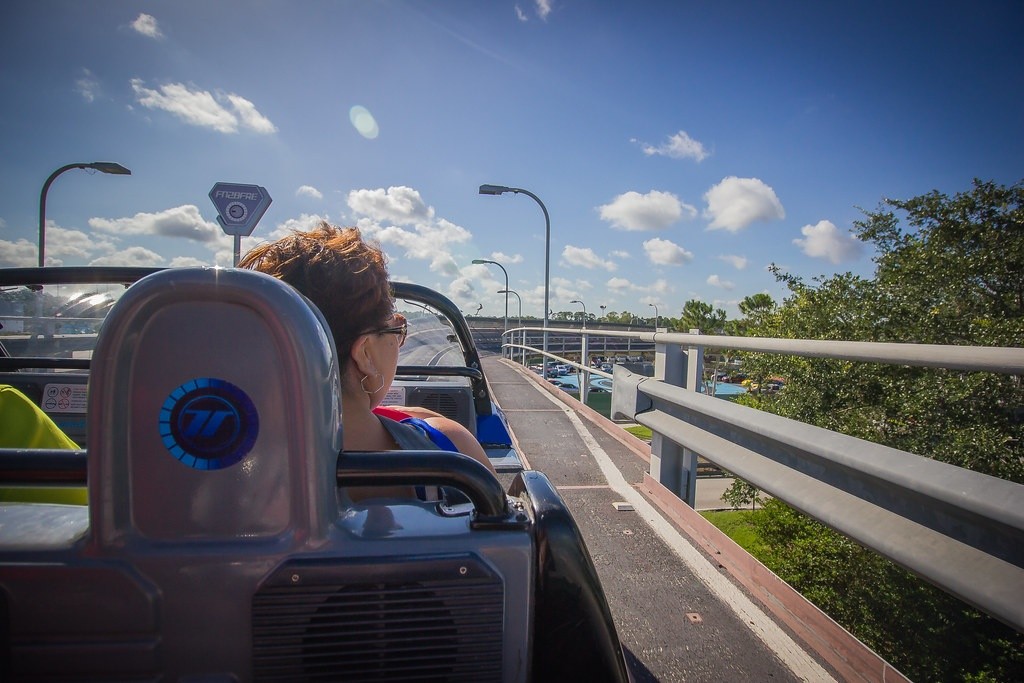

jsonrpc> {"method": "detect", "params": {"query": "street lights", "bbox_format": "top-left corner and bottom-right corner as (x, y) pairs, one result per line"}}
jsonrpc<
(601, 305), (607, 321)
(649, 303), (658, 329)
(471, 183), (550, 380)
(39, 161), (132, 266)
(570, 300), (585, 326)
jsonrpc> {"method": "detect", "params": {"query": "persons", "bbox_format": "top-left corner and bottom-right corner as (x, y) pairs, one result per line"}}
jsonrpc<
(234, 220), (505, 505)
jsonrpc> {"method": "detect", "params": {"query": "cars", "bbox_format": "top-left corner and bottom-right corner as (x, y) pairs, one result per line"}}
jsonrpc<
(705, 368), (783, 394)
(539, 355), (645, 379)
(0, 264), (629, 683)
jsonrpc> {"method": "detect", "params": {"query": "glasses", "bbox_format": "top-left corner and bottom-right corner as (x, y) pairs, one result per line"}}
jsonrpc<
(344, 313), (407, 348)
